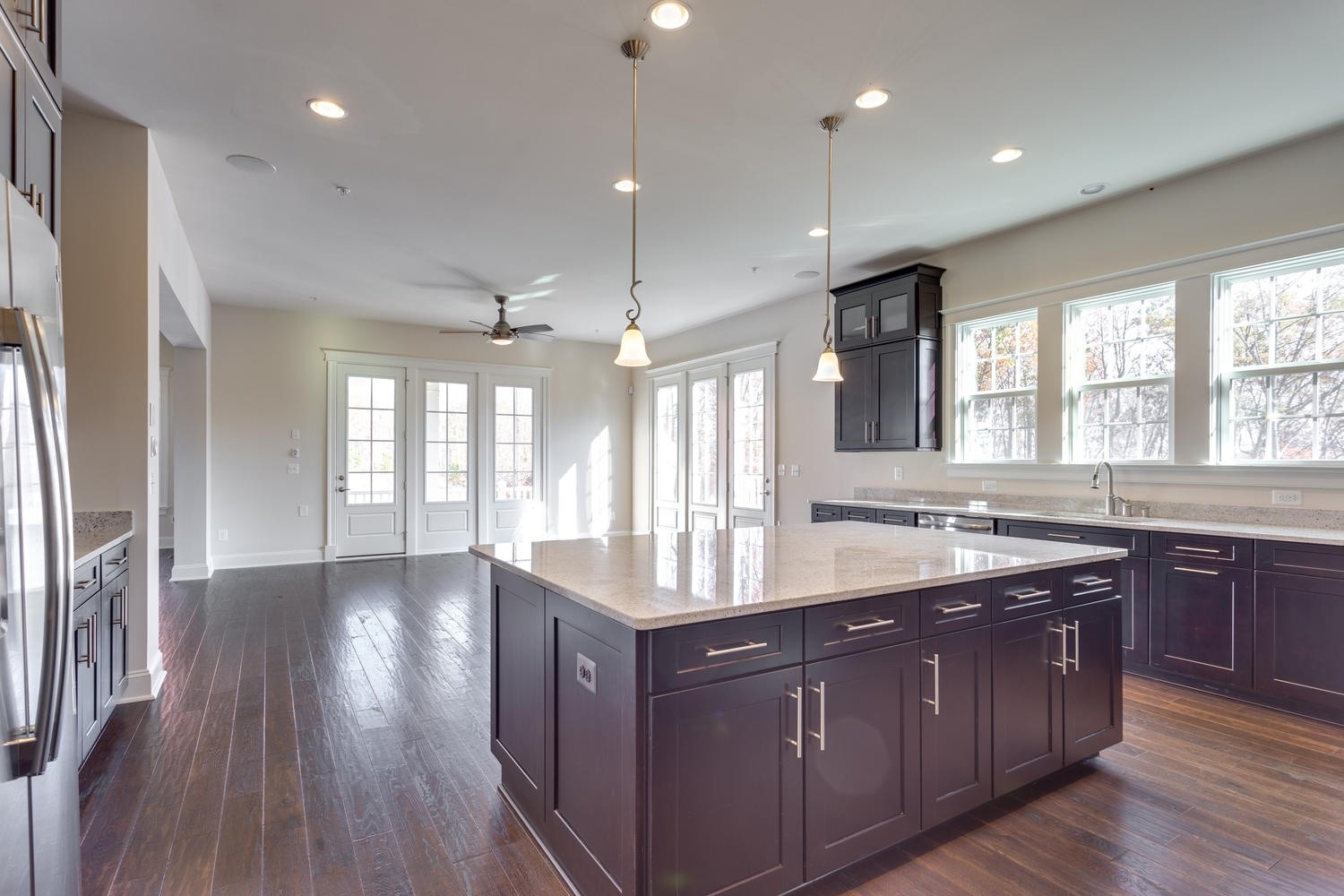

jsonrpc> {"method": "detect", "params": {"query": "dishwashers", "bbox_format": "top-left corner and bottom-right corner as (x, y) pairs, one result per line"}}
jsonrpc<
(918, 513), (994, 535)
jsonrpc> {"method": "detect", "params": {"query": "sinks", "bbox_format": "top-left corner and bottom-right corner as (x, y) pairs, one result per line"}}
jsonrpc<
(1025, 511), (1157, 523)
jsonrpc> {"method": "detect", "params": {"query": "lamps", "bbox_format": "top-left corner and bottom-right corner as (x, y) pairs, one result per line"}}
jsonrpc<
(812, 116), (845, 382)
(615, 39), (652, 367)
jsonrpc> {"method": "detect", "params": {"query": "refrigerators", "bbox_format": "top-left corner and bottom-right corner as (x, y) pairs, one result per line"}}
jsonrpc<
(0, 175), (80, 896)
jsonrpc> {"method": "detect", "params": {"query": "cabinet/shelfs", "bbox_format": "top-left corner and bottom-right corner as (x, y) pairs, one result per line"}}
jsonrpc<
(0, 0), (63, 244)
(74, 537), (129, 770)
(810, 505), (1344, 728)
(827, 262), (943, 453)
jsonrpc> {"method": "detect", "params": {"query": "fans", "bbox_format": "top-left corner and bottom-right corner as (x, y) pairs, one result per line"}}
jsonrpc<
(438, 294), (554, 346)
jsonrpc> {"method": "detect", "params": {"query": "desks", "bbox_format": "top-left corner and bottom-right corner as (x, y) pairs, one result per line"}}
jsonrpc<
(467, 521), (1128, 896)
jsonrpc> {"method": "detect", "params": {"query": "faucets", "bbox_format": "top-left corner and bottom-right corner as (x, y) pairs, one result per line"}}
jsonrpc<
(1090, 459), (1126, 516)
